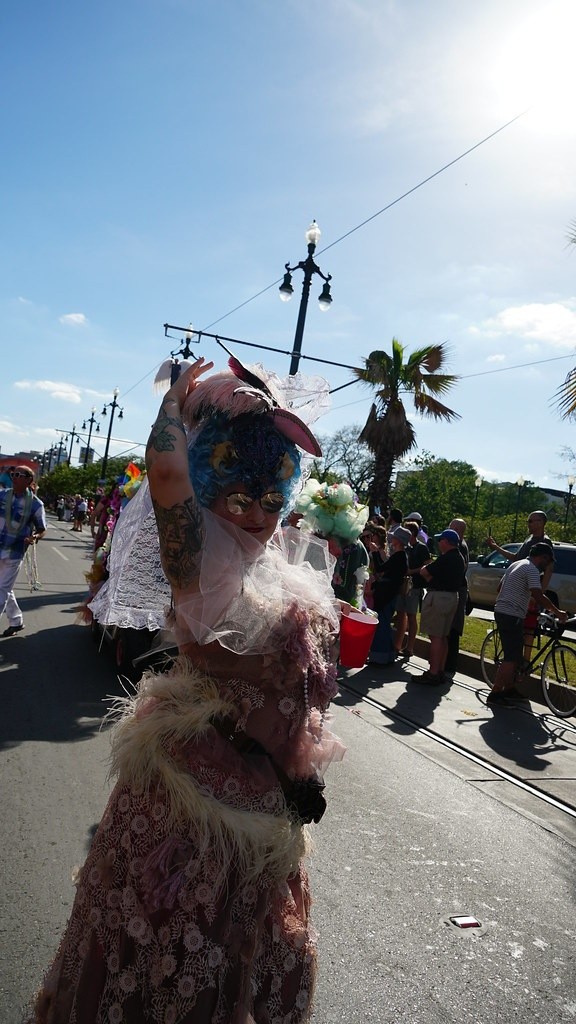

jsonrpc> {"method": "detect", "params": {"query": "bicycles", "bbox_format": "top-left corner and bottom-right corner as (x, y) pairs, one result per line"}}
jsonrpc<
(479, 613), (576, 718)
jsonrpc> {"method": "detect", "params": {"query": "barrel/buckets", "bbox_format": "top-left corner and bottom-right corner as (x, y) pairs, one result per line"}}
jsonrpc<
(337, 611), (380, 668)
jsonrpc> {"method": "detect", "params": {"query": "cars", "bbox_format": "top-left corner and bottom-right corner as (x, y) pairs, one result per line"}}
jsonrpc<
(466, 541), (576, 636)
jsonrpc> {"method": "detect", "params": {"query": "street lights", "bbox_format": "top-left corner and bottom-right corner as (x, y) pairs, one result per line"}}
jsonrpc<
(468, 478), (482, 546)
(100, 385), (125, 488)
(511, 475), (525, 542)
(278, 217), (333, 410)
(78, 405), (101, 495)
(30, 433), (67, 477)
(562, 475), (576, 540)
(65, 423), (80, 467)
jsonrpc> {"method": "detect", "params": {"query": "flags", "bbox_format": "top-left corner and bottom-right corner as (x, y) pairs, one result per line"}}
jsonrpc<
(119, 462), (141, 484)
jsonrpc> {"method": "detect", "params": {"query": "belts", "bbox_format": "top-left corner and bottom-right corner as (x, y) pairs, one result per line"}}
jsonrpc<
(427, 589), (457, 592)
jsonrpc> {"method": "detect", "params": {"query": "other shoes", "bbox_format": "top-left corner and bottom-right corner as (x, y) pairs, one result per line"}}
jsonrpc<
(3, 624), (25, 636)
(502, 688), (529, 702)
(398, 648), (413, 656)
(439, 673), (446, 683)
(487, 694), (516, 709)
(411, 670), (439, 686)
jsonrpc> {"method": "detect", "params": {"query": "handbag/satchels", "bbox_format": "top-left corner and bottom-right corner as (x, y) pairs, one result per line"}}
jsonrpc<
(209, 715), (326, 825)
(78, 505), (86, 512)
(401, 554), (413, 597)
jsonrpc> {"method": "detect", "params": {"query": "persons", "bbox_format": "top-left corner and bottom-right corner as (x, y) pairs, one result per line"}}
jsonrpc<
(484, 509), (569, 709)
(359, 507), (468, 686)
(0, 464), (47, 637)
(24, 354), (342, 1023)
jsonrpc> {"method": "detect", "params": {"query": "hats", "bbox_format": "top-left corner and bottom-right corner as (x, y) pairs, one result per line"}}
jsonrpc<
(532, 542), (556, 562)
(404, 512), (422, 521)
(387, 526), (412, 545)
(434, 529), (458, 543)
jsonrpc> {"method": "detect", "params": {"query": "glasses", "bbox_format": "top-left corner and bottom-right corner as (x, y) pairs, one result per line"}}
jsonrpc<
(361, 534), (371, 539)
(438, 538), (444, 542)
(528, 520), (541, 523)
(220, 492), (285, 515)
(10, 471), (28, 478)
